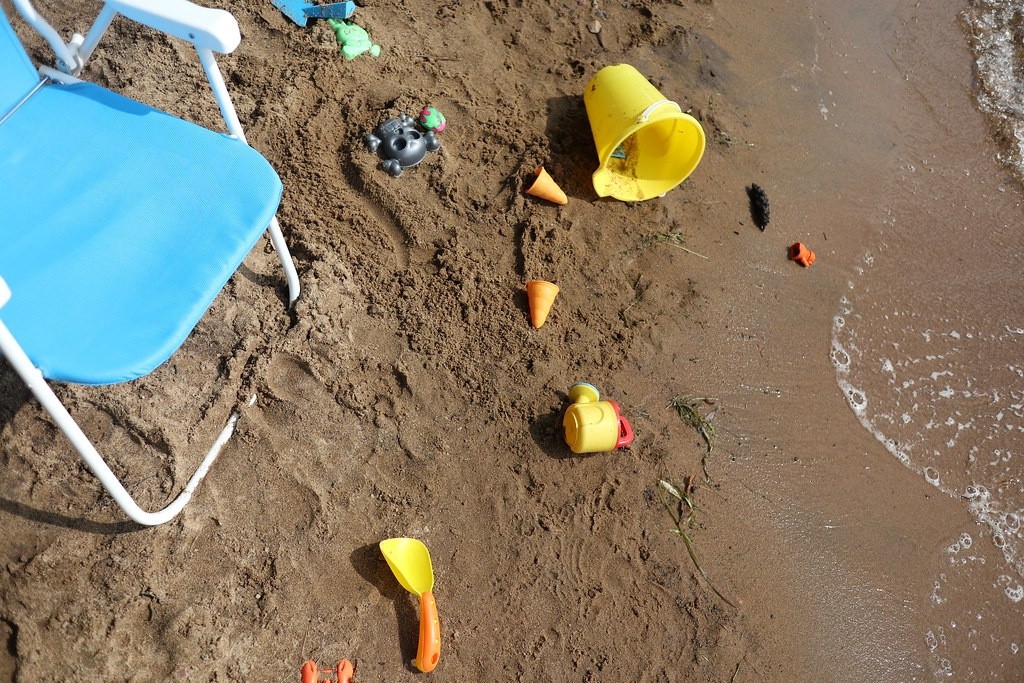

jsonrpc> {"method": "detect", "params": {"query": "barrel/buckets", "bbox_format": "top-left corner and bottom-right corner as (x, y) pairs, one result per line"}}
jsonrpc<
(583, 63), (705, 202)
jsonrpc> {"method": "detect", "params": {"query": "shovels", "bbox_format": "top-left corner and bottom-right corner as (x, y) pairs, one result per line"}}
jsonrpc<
(271, 0), (356, 27)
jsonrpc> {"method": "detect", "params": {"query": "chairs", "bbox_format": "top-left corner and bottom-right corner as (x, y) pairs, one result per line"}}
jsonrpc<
(0, 0), (301, 526)
